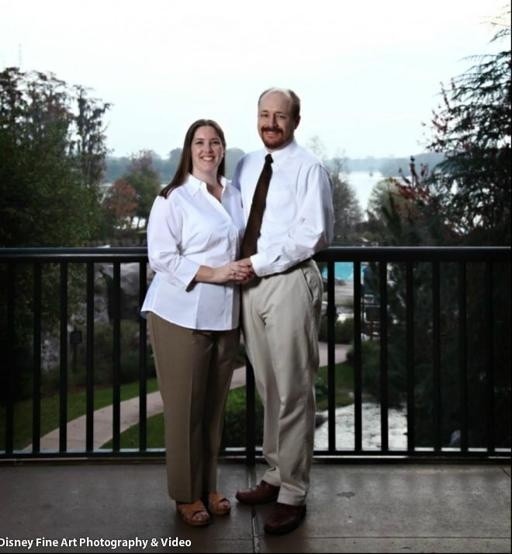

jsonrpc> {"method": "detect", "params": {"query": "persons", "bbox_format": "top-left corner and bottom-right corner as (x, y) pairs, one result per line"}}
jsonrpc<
(140, 87), (334, 535)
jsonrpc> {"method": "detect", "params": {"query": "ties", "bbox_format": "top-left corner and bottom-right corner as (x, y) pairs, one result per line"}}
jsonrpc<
(238, 152), (274, 259)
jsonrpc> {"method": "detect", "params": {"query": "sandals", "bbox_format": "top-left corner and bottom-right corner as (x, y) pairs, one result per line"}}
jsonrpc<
(176, 495), (211, 526)
(203, 489), (232, 516)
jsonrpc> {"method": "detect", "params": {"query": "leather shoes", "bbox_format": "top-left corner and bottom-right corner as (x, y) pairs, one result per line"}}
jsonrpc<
(235, 479), (280, 504)
(263, 500), (306, 535)
